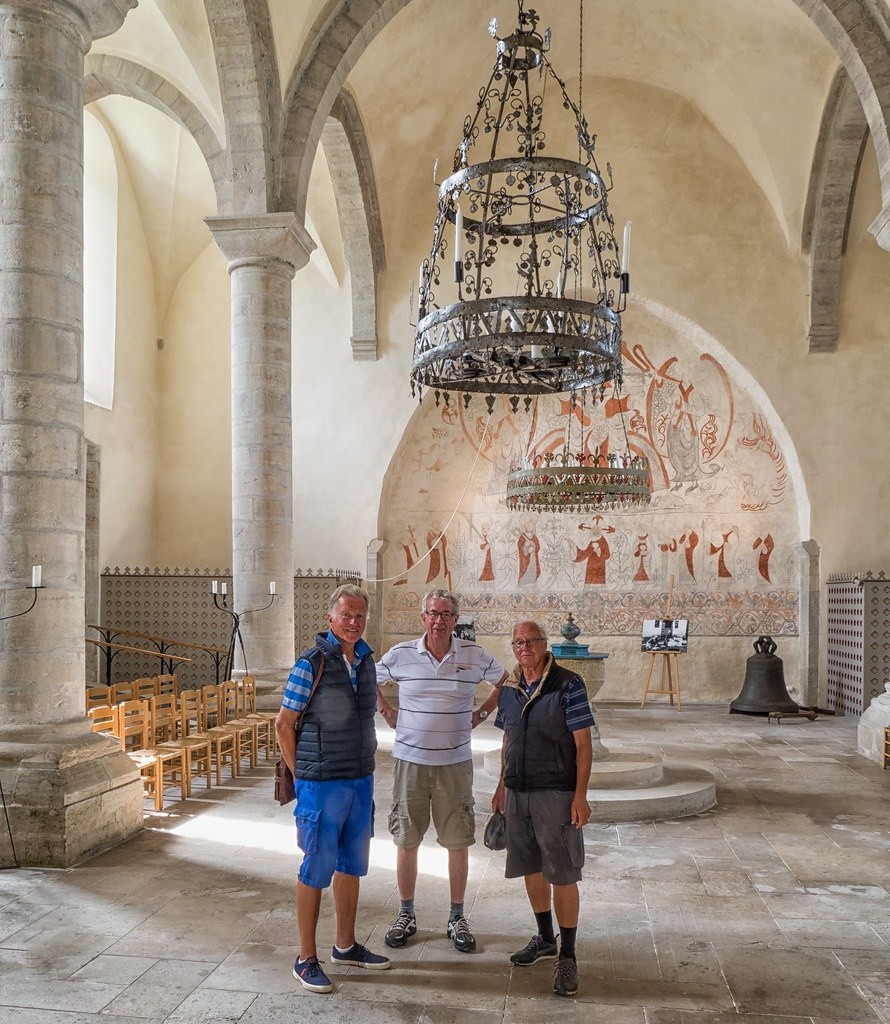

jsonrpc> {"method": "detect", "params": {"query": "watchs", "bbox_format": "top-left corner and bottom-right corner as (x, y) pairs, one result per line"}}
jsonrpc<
(479, 709), (488, 720)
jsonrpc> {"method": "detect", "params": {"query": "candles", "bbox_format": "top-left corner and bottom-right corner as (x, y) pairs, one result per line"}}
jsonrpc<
(222, 582), (227, 594)
(211, 580), (217, 594)
(32, 564), (42, 588)
(270, 581), (276, 593)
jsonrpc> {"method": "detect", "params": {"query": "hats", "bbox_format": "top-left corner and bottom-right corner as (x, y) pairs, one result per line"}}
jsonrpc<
(484, 810), (507, 851)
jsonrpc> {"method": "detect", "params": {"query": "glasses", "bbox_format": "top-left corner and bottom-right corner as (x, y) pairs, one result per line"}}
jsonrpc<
(426, 610), (455, 621)
(513, 638), (545, 648)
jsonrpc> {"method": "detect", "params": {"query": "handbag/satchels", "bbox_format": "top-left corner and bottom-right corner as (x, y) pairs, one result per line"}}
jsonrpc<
(274, 757), (295, 806)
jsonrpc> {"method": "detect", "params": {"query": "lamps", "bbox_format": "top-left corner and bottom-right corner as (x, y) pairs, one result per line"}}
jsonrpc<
(408, 0), (633, 410)
(507, 385), (652, 514)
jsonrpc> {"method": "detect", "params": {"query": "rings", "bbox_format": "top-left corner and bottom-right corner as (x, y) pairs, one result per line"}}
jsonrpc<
(587, 819), (591, 821)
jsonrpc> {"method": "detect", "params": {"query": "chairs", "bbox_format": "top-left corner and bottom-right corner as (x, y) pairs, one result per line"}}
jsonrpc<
(86, 667), (279, 812)
(882, 726), (890, 769)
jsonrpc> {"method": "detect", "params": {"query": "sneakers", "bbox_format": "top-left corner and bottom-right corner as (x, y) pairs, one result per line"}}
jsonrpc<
(292, 956), (333, 993)
(331, 942), (391, 970)
(510, 934), (560, 966)
(553, 953), (579, 995)
(384, 911), (417, 947)
(446, 915), (476, 951)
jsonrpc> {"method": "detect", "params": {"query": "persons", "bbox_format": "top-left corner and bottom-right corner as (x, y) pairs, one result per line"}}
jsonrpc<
(275, 584), (390, 993)
(375, 590), (509, 950)
(491, 621), (595, 996)
